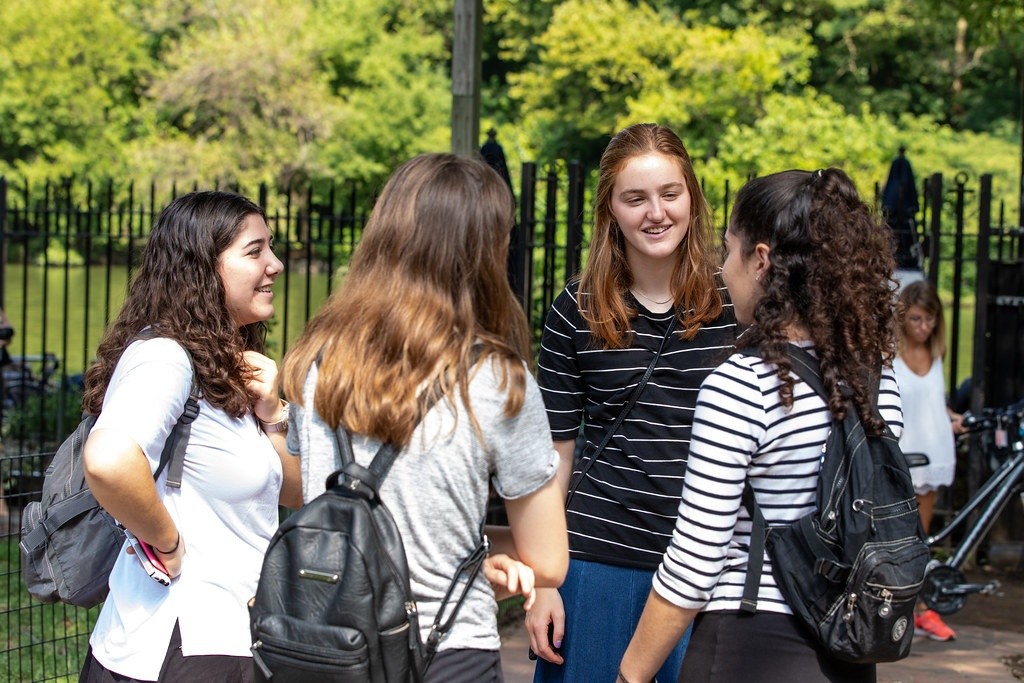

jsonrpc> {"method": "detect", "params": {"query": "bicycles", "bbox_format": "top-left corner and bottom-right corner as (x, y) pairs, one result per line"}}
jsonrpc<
(899, 400), (1023, 615)
(0, 355), (59, 424)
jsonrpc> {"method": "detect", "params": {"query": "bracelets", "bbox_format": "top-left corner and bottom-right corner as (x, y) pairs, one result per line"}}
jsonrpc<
(260, 399), (289, 432)
(618, 667), (628, 683)
(155, 533), (180, 554)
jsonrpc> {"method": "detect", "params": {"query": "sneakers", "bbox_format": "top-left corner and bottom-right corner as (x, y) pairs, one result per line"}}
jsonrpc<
(912, 610), (957, 641)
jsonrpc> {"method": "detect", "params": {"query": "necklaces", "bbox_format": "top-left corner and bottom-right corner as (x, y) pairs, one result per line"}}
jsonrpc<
(633, 287), (673, 304)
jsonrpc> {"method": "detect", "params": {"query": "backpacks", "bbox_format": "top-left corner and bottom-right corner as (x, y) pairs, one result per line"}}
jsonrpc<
(730, 340), (930, 664)
(18, 330), (199, 611)
(247, 344), (497, 683)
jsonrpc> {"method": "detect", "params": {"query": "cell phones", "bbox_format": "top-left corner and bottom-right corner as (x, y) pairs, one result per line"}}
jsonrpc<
(114, 519), (171, 587)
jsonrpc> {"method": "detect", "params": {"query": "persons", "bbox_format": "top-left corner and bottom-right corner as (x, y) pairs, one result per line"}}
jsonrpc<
(287, 152), (569, 682)
(76, 195), (303, 682)
(889, 280), (966, 641)
(520, 123), (753, 683)
(612, 169), (903, 683)
